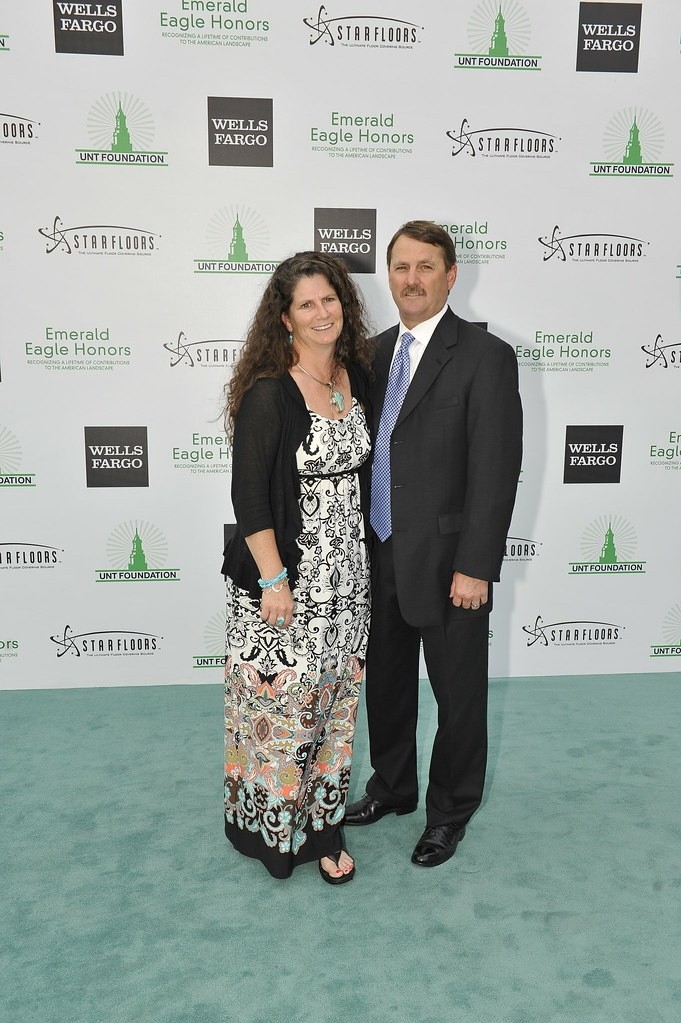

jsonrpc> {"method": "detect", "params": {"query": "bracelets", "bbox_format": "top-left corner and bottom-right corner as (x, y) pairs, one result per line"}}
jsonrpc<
(257, 566), (290, 595)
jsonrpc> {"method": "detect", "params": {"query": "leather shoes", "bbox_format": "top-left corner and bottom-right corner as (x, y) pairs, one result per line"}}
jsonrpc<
(341, 794), (419, 826)
(411, 820), (466, 866)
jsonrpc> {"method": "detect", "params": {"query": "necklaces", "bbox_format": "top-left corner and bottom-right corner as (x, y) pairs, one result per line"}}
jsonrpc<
(288, 348), (346, 413)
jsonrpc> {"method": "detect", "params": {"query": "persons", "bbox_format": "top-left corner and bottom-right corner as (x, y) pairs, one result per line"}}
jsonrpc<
(342, 218), (524, 868)
(219, 253), (382, 884)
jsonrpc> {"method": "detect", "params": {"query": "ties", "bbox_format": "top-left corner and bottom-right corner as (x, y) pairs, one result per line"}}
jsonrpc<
(369, 333), (415, 540)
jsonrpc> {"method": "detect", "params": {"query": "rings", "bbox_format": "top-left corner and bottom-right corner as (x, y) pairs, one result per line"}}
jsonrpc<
(275, 616), (285, 626)
(471, 603), (481, 608)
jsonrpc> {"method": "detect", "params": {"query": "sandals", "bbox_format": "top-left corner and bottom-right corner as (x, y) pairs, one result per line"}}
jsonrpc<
(319, 847), (357, 884)
